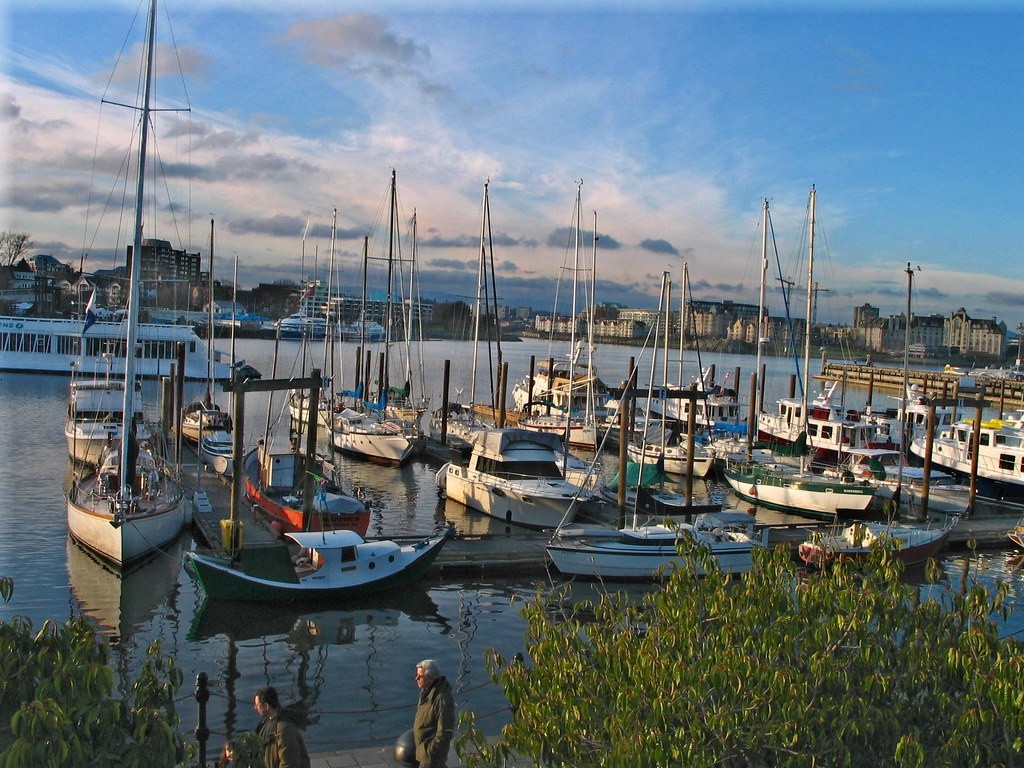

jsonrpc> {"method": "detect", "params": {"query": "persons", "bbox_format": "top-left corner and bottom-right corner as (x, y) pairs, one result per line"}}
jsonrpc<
(219, 687), (311, 768)
(414, 659), (456, 768)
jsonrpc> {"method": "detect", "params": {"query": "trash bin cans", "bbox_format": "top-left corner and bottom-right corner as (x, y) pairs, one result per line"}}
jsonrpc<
(220, 519), (244, 550)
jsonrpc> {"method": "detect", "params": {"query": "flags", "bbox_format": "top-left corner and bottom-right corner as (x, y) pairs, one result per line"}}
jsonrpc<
(81, 286), (96, 337)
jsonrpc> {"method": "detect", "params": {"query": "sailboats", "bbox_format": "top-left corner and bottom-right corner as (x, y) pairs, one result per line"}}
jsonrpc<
(1, 1), (1023, 609)
